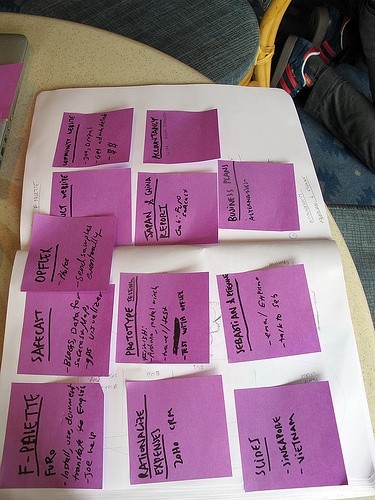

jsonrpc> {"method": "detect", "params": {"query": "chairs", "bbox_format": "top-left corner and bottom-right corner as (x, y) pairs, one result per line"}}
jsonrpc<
(0, 1), (297, 91)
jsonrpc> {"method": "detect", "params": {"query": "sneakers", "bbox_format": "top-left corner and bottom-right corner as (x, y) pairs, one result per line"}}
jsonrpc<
(271, 35), (323, 97)
(318, 11), (353, 64)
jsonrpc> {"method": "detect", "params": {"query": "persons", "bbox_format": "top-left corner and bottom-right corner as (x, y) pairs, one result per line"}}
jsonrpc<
(1, 1), (271, 86)
(273, 1), (375, 175)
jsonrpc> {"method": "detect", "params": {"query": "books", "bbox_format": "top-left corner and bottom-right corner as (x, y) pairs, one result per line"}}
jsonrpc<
(0, 78), (375, 500)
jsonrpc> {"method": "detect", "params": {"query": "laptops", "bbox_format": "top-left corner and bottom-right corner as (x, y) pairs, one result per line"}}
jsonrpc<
(1, 32), (30, 169)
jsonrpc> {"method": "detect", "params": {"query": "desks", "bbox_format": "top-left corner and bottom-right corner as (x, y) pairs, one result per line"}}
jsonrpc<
(0, 11), (375, 499)
